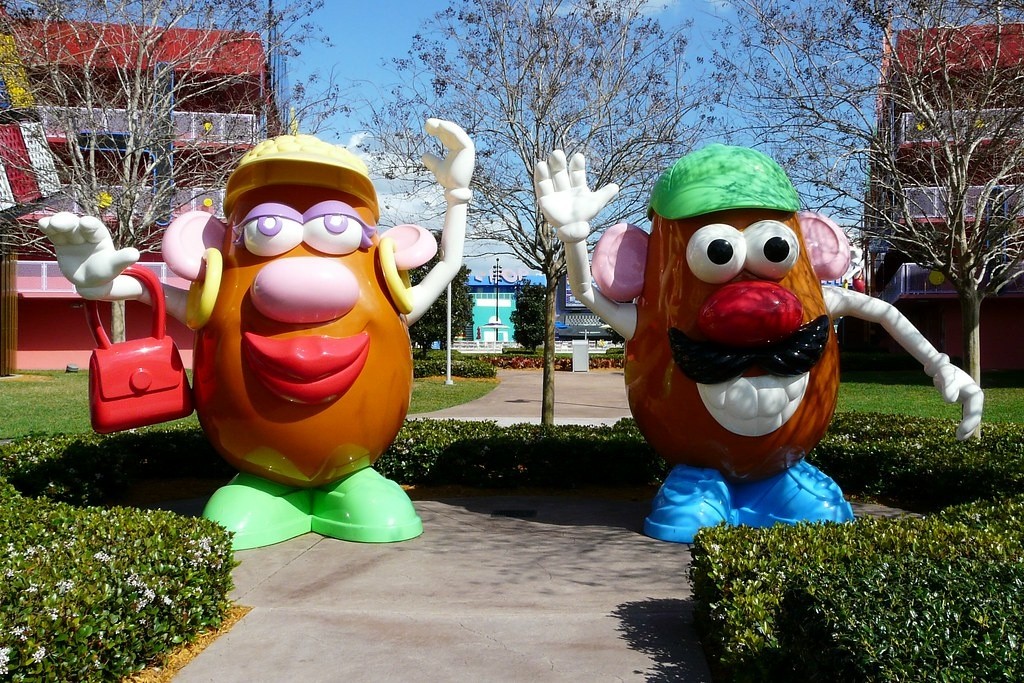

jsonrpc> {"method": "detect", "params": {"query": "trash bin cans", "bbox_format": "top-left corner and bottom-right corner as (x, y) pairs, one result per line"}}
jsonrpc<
(571, 339), (590, 373)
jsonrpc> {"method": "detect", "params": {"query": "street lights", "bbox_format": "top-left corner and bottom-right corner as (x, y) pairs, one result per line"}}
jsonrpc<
(493, 257), (502, 344)
